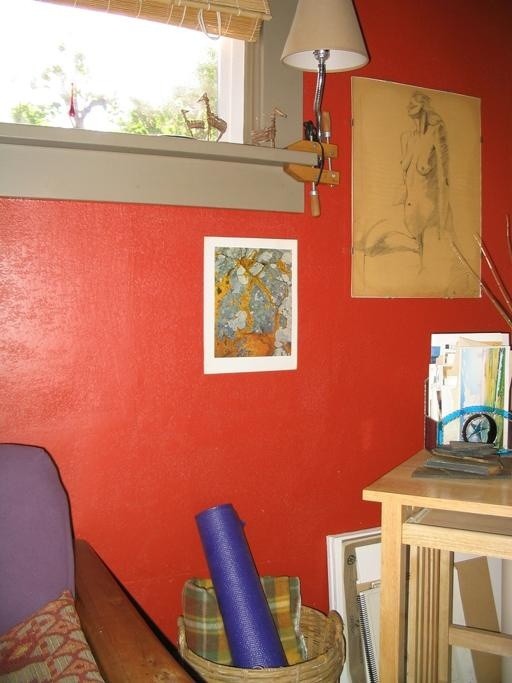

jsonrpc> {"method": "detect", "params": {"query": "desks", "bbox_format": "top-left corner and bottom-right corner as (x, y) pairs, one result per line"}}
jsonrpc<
(362, 441), (512, 683)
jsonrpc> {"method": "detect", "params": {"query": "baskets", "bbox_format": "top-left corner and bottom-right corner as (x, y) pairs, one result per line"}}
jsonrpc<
(178, 607), (346, 683)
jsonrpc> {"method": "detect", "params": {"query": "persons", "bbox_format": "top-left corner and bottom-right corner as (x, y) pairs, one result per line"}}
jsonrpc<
(358, 89), (452, 294)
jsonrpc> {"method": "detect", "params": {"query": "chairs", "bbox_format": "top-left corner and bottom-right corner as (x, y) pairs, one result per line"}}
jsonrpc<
(1, 441), (203, 683)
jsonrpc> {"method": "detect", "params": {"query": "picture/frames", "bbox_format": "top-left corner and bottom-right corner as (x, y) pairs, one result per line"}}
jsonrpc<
(200, 234), (303, 377)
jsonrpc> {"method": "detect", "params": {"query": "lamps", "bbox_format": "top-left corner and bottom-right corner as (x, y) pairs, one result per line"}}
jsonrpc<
(281, 1), (371, 146)
